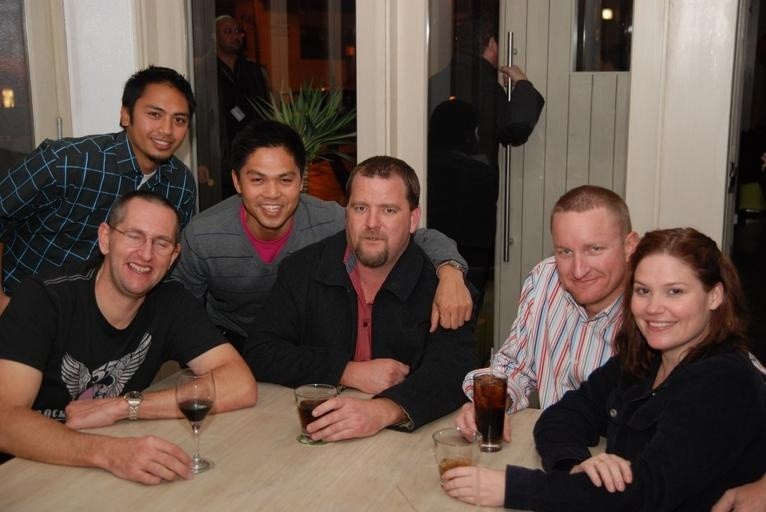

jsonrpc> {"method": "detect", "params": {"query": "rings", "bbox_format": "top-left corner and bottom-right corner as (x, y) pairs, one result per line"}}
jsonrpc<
(594, 460), (603, 467)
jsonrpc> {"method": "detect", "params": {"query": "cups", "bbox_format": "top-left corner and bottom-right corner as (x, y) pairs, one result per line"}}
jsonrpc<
(432, 429), (482, 489)
(293, 382), (338, 445)
(471, 371), (510, 453)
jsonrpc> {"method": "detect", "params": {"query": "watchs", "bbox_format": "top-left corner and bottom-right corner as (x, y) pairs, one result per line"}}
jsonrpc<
(123, 390), (144, 421)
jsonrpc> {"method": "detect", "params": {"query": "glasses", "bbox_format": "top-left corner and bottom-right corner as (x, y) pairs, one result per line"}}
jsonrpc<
(107, 223), (178, 258)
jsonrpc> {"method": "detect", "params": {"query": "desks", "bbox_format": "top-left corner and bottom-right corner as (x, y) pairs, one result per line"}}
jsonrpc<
(0, 367), (609, 512)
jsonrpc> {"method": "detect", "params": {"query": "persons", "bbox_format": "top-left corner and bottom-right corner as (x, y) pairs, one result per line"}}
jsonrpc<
(164, 120), (474, 351)
(440, 228), (766, 512)
(1, 189), (258, 486)
(425, 22), (545, 363)
(0, 64), (198, 318)
(711, 472), (766, 512)
(242, 155), (491, 443)
(456, 184), (640, 443)
(193, 15), (273, 200)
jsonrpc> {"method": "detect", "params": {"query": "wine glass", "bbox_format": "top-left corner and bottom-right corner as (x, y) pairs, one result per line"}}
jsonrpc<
(175, 366), (213, 474)
